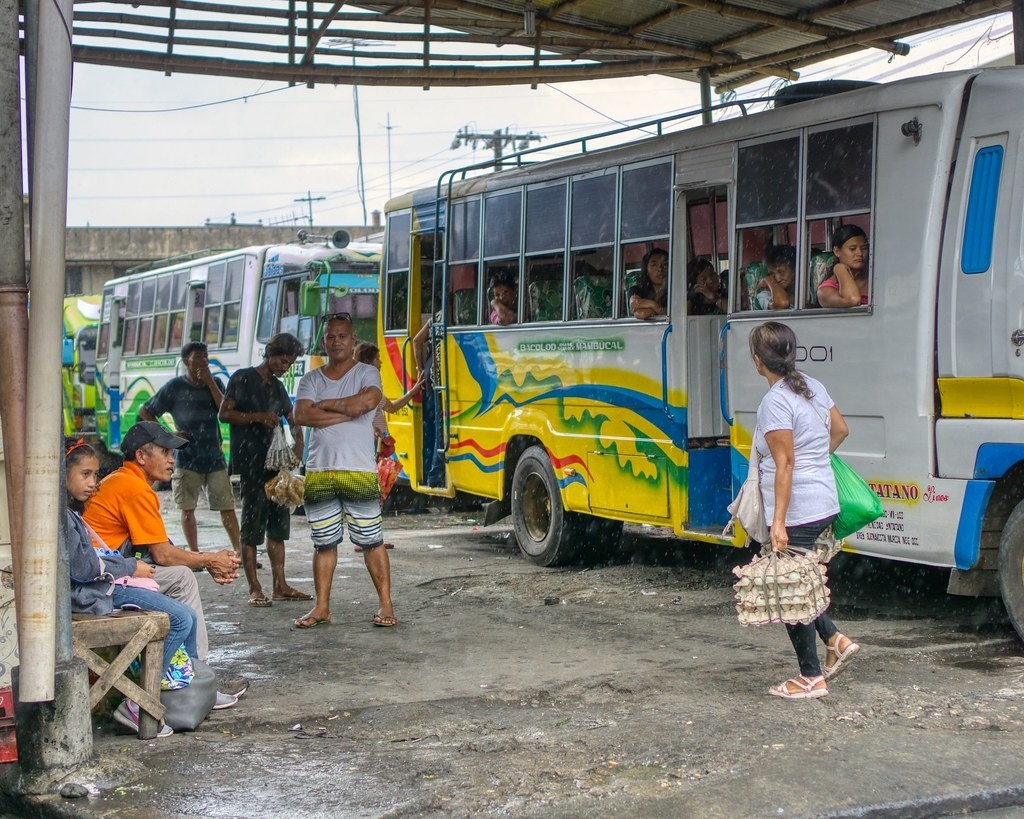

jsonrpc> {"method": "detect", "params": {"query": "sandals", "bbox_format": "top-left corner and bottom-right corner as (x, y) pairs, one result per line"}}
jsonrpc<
(822, 634), (860, 683)
(769, 674), (828, 699)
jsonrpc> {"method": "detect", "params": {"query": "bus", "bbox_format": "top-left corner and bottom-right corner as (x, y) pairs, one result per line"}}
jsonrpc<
(379, 63), (1024, 644)
(27, 229), (383, 477)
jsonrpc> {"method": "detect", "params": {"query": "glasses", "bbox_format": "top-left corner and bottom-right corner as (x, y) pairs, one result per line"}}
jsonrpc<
(321, 312), (352, 326)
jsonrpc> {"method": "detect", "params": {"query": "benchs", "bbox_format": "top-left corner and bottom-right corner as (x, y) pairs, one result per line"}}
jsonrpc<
(68, 608), (170, 740)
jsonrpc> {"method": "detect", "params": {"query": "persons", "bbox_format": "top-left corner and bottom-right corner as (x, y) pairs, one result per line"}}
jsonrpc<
(752, 244), (796, 308)
(139, 341), (262, 571)
(627, 247), (667, 319)
(490, 273), (518, 325)
(354, 336), (426, 552)
(83, 421), (250, 698)
(817, 223), (869, 306)
(292, 312), (397, 628)
(219, 333), (313, 605)
(412, 310), (470, 488)
(64, 437), (238, 737)
(749, 322), (859, 699)
(687, 257), (728, 316)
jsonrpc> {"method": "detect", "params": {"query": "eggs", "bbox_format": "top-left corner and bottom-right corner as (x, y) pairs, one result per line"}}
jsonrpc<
(739, 572), (826, 614)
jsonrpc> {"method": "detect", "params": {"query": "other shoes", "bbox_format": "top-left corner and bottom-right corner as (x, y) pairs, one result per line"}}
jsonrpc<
(189, 566), (203, 573)
(235, 555), (263, 569)
(354, 541), (395, 551)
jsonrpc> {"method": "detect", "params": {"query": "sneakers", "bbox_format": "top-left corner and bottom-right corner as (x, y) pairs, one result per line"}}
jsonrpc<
(216, 678), (248, 696)
(113, 698), (174, 737)
(212, 691), (238, 709)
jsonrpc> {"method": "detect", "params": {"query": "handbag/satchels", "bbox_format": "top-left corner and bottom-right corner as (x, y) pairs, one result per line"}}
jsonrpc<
(121, 641), (194, 690)
(722, 477), (770, 544)
(265, 470), (307, 507)
(830, 452), (883, 540)
(109, 574), (160, 593)
(265, 424), (301, 470)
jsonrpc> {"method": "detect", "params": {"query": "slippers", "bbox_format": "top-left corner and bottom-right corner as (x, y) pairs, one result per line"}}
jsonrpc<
(297, 614), (329, 628)
(271, 590), (312, 600)
(374, 615), (396, 626)
(249, 596), (271, 607)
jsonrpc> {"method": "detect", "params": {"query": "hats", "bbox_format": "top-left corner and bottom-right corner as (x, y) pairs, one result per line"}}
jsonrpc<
(121, 421), (190, 454)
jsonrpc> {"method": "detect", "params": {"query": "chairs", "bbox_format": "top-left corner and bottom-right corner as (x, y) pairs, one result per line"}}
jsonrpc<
(394, 278), (449, 328)
(453, 270), (648, 327)
(747, 252), (839, 311)
